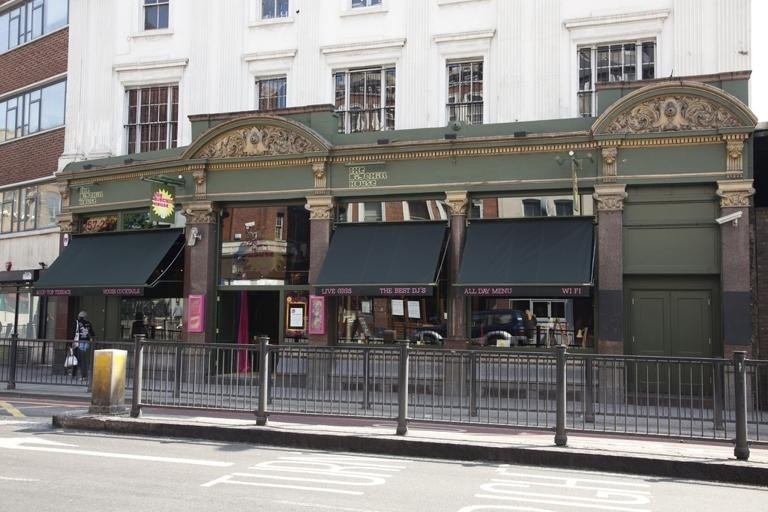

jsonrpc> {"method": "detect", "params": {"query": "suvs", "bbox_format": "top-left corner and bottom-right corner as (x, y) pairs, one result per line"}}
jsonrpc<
(412, 310), (536, 349)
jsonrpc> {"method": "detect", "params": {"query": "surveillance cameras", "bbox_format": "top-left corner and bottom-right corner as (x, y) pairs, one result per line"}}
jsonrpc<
(245, 222), (255, 230)
(715, 210), (743, 226)
(187, 227), (201, 246)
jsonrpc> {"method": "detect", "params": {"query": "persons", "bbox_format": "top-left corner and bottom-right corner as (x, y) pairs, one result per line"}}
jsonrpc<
(65, 311), (96, 381)
(130, 301), (183, 340)
